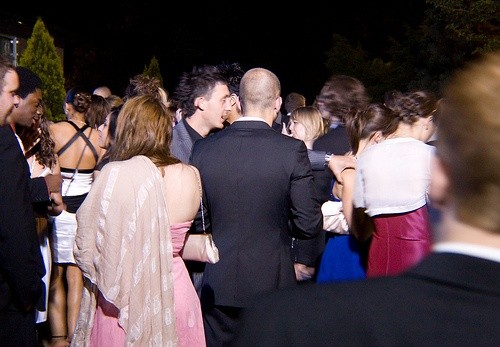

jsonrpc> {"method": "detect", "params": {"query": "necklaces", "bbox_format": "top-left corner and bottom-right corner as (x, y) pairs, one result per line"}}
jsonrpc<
(67, 119), (88, 125)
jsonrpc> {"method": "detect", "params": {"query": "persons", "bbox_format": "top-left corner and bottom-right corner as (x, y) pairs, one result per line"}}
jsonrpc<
(94, 57), (444, 282)
(0, 66), (63, 347)
(166, 68), (231, 165)
(229, 51), (500, 347)
(190, 68), (324, 346)
(69, 97), (206, 347)
(0, 63), (19, 126)
(46, 88), (101, 347)
(227, 262), (418, 347)
(18, 100), (64, 324)
(354, 92), (418, 276)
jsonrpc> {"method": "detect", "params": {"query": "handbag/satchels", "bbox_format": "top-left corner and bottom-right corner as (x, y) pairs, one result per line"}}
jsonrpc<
(178, 234), (219, 264)
(320, 201), (351, 235)
(348, 207), (375, 252)
